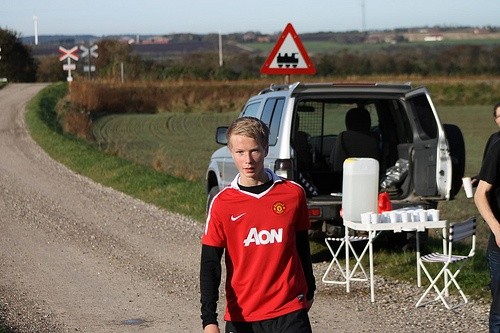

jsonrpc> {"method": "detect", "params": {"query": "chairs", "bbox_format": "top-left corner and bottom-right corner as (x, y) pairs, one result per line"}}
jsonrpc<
(331, 108), (388, 163)
(322, 191), (389, 284)
(415, 216), (478, 308)
(295, 114), (312, 147)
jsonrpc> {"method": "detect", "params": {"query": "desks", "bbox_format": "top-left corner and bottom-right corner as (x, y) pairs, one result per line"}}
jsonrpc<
(342, 218), (448, 302)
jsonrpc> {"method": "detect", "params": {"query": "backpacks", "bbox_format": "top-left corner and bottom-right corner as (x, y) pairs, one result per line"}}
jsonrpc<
(297, 162), (325, 198)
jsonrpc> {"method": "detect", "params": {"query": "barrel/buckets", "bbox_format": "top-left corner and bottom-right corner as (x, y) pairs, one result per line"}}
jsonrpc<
(342, 156), (379, 222)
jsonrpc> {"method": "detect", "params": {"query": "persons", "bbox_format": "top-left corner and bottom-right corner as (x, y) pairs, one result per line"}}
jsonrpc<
(462, 103), (500, 292)
(474, 139), (500, 333)
(199, 118), (316, 333)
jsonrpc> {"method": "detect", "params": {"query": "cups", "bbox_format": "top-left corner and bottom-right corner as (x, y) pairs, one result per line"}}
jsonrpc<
(360, 207), (441, 224)
(462, 177), (473, 198)
